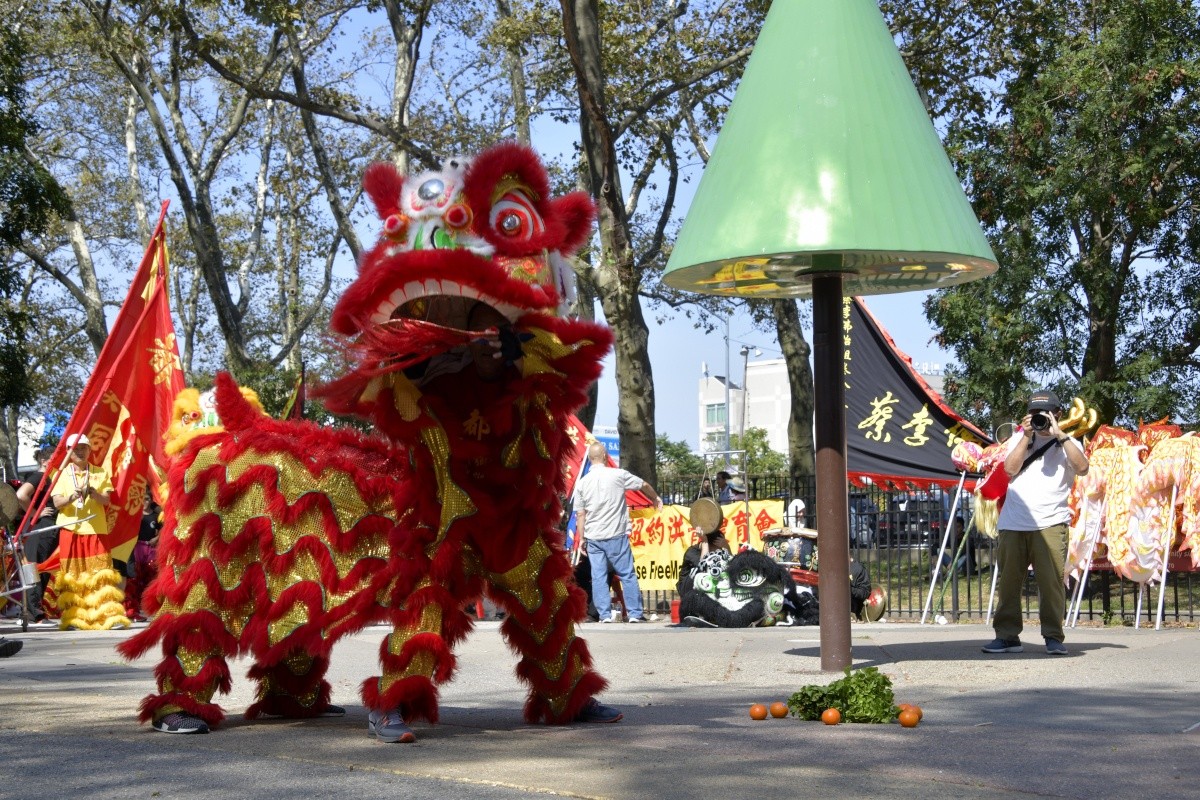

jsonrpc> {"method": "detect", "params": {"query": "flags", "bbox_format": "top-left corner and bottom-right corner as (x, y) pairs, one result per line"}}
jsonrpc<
(37, 224), (188, 574)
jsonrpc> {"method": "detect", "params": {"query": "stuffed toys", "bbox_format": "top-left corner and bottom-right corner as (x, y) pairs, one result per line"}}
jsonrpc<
(679, 543), (819, 628)
(157, 385), (274, 526)
(118, 146), (625, 735)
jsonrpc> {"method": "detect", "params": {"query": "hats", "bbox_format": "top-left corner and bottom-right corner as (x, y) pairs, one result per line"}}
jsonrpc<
(726, 477), (746, 492)
(1028, 391), (1060, 413)
(66, 433), (91, 450)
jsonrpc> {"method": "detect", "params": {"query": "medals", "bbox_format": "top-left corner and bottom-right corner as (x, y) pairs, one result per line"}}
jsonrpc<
(77, 502), (83, 509)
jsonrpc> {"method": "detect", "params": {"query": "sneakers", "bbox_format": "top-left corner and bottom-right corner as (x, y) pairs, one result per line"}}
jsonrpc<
(152, 711), (209, 734)
(981, 638), (1023, 653)
(368, 707), (416, 743)
(540, 697), (623, 724)
(1046, 636), (1068, 654)
(259, 692), (346, 720)
(17, 618), (59, 627)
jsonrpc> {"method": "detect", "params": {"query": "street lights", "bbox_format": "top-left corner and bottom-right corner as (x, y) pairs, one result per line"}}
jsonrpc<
(738, 345), (762, 476)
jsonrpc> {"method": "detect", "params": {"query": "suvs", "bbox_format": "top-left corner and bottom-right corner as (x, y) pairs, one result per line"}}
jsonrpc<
(877, 492), (975, 554)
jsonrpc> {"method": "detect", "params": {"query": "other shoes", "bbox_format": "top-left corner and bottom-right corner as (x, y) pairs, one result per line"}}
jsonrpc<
(132, 615), (147, 621)
(6, 605), (21, 618)
(110, 623), (124, 630)
(496, 612), (506, 619)
(599, 616), (612, 623)
(0, 637), (23, 657)
(67, 625), (79, 630)
(629, 616), (647, 622)
(584, 614), (600, 622)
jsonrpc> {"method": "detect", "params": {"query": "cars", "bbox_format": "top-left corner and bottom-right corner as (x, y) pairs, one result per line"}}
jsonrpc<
(768, 493), (882, 546)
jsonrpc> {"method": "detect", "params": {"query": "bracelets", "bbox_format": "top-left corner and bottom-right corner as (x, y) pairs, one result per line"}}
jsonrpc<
(1060, 435), (1071, 443)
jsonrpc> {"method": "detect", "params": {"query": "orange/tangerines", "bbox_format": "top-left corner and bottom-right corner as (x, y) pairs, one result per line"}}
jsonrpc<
(770, 701), (788, 717)
(897, 702), (922, 727)
(822, 708), (840, 725)
(749, 704), (767, 719)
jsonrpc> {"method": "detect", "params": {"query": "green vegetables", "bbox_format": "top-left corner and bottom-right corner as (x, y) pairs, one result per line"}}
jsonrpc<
(787, 664), (902, 724)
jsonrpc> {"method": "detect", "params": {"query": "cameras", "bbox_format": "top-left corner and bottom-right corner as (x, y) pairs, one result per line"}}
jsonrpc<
(1030, 414), (1051, 430)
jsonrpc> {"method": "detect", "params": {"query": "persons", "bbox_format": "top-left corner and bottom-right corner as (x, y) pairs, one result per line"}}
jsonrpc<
(673, 526), (732, 599)
(136, 627), (346, 733)
(366, 302), (625, 743)
(0, 432), (165, 659)
(783, 499), (808, 528)
(692, 477), (713, 501)
(574, 442), (663, 623)
(925, 516), (977, 581)
(981, 391), (1090, 655)
(716, 471), (750, 505)
(850, 549), (872, 623)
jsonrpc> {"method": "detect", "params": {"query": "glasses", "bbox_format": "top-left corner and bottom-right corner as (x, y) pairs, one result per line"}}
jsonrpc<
(144, 497), (152, 502)
(716, 478), (723, 480)
